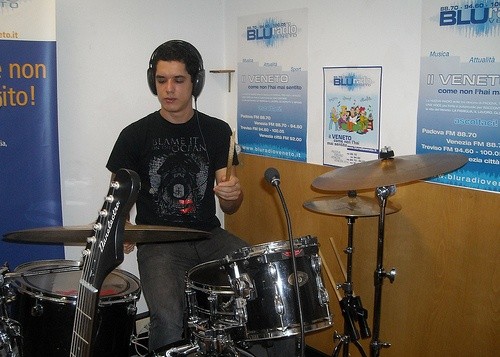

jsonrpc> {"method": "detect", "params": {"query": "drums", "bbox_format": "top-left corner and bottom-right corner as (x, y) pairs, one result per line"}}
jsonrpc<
(0, 267), (23, 357)
(0, 258), (143, 357)
(144, 340), (256, 357)
(221, 235), (335, 343)
(184, 257), (255, 352)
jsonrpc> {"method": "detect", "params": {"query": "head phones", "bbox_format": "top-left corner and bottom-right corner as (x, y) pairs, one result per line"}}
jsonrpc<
(147, 40), (205, 98)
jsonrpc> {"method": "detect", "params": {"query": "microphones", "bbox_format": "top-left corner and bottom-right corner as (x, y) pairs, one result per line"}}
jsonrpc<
(264, 167), (306, 357)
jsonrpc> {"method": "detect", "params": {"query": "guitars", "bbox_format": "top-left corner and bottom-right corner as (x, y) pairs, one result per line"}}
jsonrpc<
(70, 167), (142, 357)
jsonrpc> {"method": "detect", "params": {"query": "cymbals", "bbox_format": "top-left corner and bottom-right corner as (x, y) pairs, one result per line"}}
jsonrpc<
(311, 152), (468, 192)
(302, 193), (401, 217)
(0, 218), (215, 244)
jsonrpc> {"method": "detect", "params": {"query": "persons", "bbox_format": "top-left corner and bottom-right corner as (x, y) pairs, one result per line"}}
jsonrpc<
(106, 41), (297, 357)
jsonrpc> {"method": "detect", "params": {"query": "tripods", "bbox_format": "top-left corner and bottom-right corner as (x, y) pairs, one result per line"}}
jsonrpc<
(331, 217), (368, 357)
